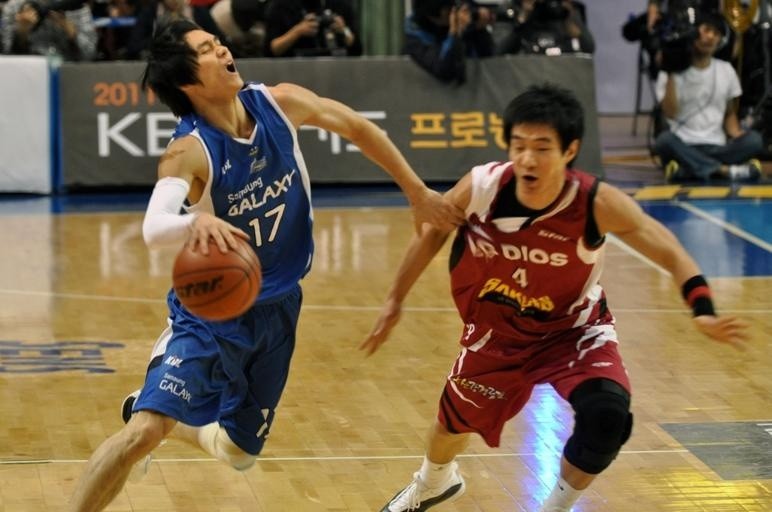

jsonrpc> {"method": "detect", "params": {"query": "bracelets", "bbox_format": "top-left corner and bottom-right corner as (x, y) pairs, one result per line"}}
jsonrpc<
(679, 273), (720, 319)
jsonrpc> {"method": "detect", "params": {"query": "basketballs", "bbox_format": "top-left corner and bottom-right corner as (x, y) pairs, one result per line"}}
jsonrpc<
(173, 233), (262, 322)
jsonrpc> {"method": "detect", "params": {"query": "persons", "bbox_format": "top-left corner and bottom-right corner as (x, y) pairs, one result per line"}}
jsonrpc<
(399, 1), (594, 86)
(356, 78), (754, 512)
(619, 0), (771, 186)
(1, 2), (364, 62)
(65, 17), (469, 512)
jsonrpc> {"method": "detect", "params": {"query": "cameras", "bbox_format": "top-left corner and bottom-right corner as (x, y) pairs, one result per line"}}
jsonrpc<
(313, 13), (333, 28)
(458, 0), (502, 20)
(19, 0), (85, 29)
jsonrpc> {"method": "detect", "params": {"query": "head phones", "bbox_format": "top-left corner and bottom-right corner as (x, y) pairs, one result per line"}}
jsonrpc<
(718, 17), (732, 49)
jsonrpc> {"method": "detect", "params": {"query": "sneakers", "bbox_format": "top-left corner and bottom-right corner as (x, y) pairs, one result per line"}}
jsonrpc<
(664, 159), (681, 182)
(121, 390), (141, 423)
(741, 159), (762, 182)
(380, 463), (464, 512)
